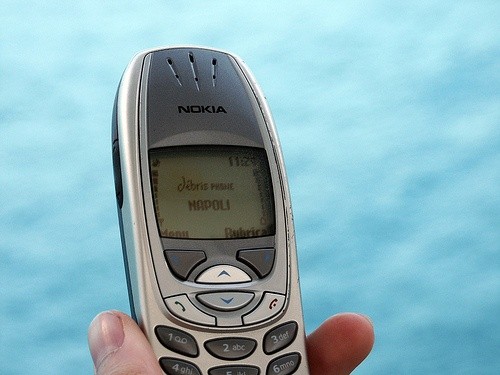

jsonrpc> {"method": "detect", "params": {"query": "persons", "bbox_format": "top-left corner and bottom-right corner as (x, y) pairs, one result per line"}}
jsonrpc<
(86, 310), (376, 375)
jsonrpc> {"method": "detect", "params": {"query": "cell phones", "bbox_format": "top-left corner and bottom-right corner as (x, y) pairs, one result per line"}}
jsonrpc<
(111, 46), (310, 375)
